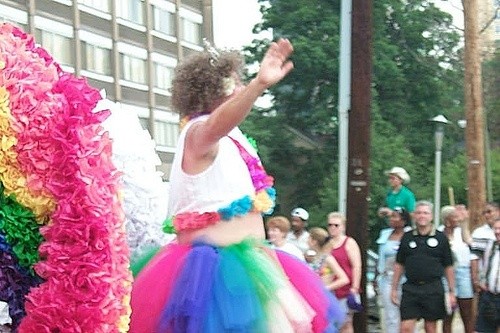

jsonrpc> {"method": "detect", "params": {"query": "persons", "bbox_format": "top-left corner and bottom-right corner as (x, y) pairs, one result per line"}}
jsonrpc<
(129, 37), (352, 333)
(265, 208), (363, 333)
(374, 166), (417, 227)
(370, 200), (500, 333)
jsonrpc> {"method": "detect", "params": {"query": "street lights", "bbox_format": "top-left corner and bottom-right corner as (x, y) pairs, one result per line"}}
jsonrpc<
(427, 114), (453, 229)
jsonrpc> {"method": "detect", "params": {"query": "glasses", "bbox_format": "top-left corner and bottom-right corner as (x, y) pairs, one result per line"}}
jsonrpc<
(328, 224), (341, 227)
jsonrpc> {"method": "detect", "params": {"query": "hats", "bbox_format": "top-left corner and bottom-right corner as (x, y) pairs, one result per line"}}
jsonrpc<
(385, 166), (410, 183)
(291, 207), (309, 220)
